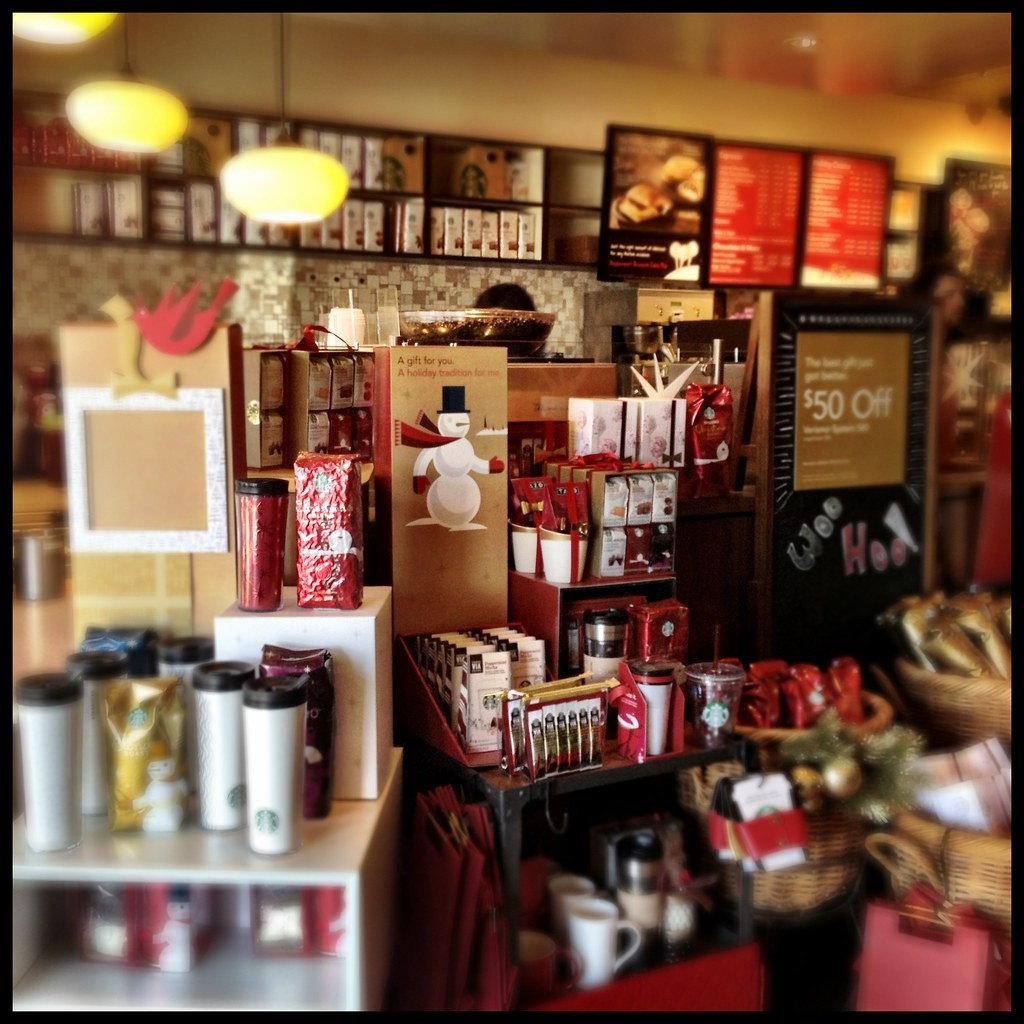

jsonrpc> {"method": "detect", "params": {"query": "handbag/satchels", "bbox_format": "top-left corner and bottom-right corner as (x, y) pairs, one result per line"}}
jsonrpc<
(845, 893), (1009, 1011)
(392, 783), (518, 1014)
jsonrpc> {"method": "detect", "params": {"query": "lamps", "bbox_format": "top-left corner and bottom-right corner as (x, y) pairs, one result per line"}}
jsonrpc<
(64, 14), (189, 154)
(217, 13), (352, 227)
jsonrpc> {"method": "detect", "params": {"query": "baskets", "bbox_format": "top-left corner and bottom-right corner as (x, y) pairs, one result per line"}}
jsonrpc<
(734, 690), (894, 773)
(865, 806), (1011, 947)
(680, 763), (866, 923)
(895, 654), (1010, 751)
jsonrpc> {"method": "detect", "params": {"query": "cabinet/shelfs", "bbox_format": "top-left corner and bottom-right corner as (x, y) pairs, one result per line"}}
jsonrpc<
(391, 717), (753, 1000)
(12, 747), (403, 1011)
(8, 88), (606, 268)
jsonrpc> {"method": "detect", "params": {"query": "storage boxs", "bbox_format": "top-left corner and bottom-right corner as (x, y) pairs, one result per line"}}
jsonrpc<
(567, 396), (686, 467)
(211, 583), (393, 798)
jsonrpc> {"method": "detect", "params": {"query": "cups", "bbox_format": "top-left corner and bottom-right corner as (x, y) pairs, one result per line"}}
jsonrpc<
(192, 662), (256, 832)
(685, 664), (748, 752)
(569, 899), (641, 989)
(15, 675), (85, 852)
(153, 635), (217, 815)
(540, 524), (588, 584)
(240, 676), (314, 856)
(66, 651), (130, 815)
(232, 477), (288, 613)
(507, 517), (540, 573)
(548, 874), (595, 932)
(516, 931), (582, 1001)
(12, 528), (67, 600)
(327, 307), (366, 349)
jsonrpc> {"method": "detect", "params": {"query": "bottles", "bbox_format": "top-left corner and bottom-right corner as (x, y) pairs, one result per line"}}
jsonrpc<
(582, 609), (630, 685)
(624, 659), (675, 756)
(590, 706), (602, 766)
(579, 708), (590, 767)
(510, 707), (525, 772)
(557, 712), (570, 773)
(615, 833), (665, 948)
(545, 712), (558, 773)
(531, 719), (546, 778)
(568, 710), (580, 770)
